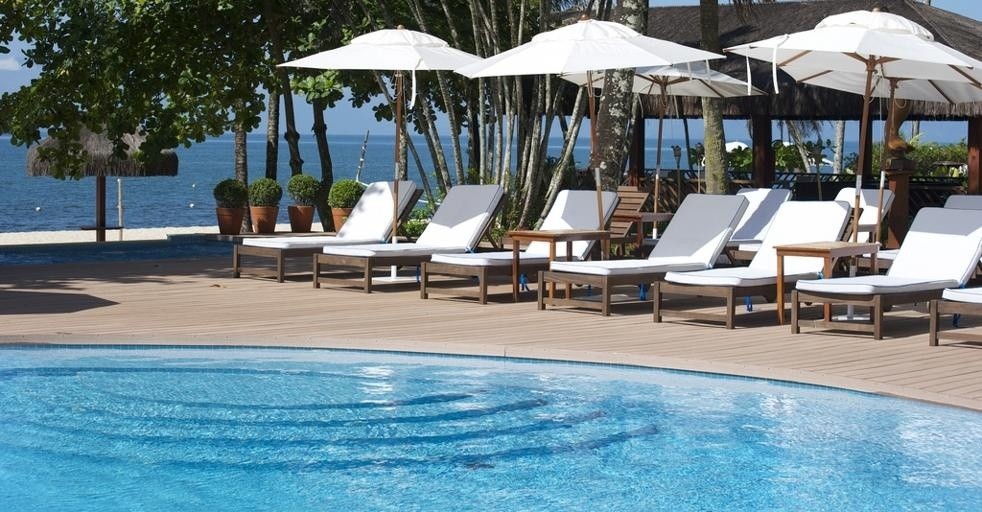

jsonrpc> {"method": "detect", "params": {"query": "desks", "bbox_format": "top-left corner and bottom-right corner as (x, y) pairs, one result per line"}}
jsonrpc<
(771, 240), (881, 325)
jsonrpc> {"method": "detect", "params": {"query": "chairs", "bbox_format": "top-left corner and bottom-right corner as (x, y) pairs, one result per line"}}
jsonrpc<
(834, 187), (895, 247)
(927, 286), (982, 346)
(232, 181), (426, 281)
(651, 199), (863, 328)
(789, 206), (980, 342)
(419, 186), (621, 304)
(537, 190), (751, 315)
(312, 184), (516, 293)
(727, 187), (794, 261)
(945, 193), (982, 210)
(605, 183), (651, 243)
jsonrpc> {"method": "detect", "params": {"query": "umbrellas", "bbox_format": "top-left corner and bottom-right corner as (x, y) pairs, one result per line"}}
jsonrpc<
(723, 20), (982, 322)
(452, 19), (728, 260)
(555, 61), (767, 240)
(274, 24), (485, 276)
(774, 7), (982, 244)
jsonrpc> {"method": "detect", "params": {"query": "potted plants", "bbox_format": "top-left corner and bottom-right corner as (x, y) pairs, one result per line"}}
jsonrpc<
(324, 179), (364, 233)
(213, 178), (247, 237)
(246, 178), (286, 234)
(286, 176), (323, 233)
